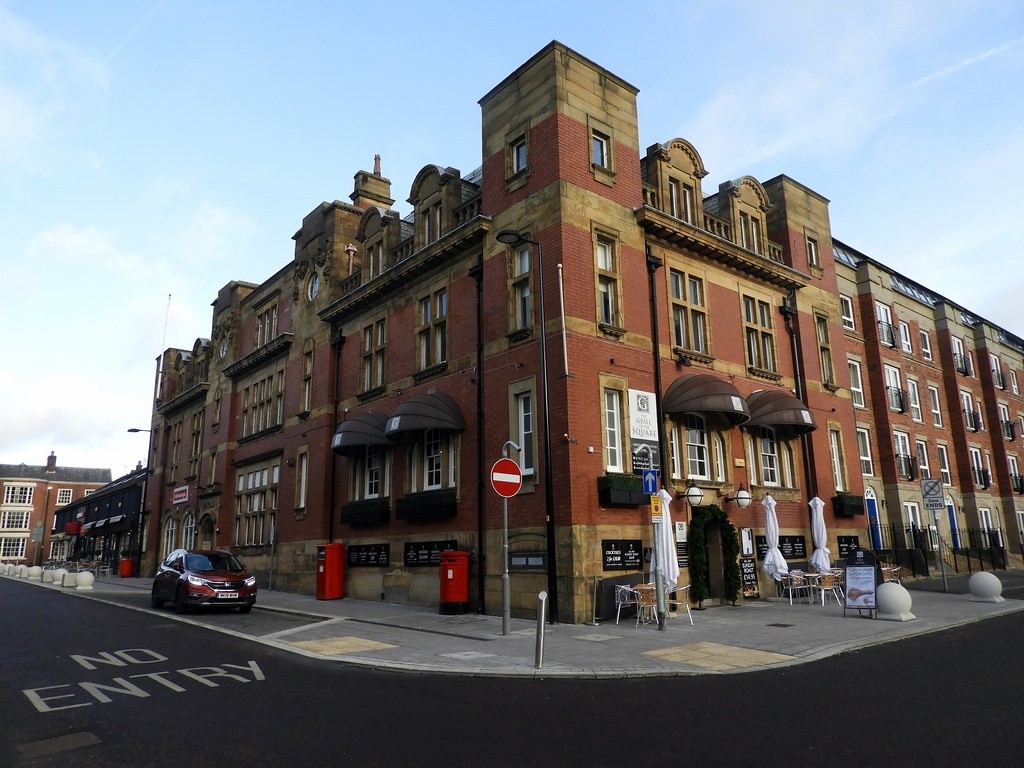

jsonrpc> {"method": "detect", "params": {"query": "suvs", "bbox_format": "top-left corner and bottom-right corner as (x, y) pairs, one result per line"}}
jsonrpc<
(151, 547), (258, 615)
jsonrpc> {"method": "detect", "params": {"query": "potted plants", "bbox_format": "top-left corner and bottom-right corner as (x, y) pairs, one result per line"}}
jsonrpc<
(66, 548), (131, 560)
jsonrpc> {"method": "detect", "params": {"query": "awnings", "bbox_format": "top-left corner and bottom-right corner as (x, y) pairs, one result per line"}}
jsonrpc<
(109, 514), (126, 523)
(96, 518), (109, 527)
(745, 388), (818, 442)
(330, 411), (390, 456)
(83, 521), (97, 529)
(661, 373), (751, 430)
(384, 393), (466, 442)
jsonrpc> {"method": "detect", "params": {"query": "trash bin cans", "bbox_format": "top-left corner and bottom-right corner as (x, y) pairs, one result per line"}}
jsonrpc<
(316, 543), (345, 601)
(118, 559), (134, 578)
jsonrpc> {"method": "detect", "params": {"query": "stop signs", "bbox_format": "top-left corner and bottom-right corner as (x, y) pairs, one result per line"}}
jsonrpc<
(491, 458), (522, 498)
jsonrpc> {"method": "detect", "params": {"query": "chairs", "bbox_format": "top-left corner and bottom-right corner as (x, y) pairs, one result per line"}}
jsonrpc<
(878, 560), (903, 587)
(611, 580), (697, 629)
(41, 559), (113, 579)
(779, 566), (845, 608)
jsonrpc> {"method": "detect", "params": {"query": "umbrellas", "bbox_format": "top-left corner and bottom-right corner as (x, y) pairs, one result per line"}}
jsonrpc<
(650, 488), (680, 597)
(761, 492), (789, 598)
(808, 494), (831, 573)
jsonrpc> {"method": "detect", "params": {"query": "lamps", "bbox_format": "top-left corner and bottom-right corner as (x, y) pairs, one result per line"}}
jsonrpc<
(675, 478), (705, 507)
(722, 481), (753, 509)
